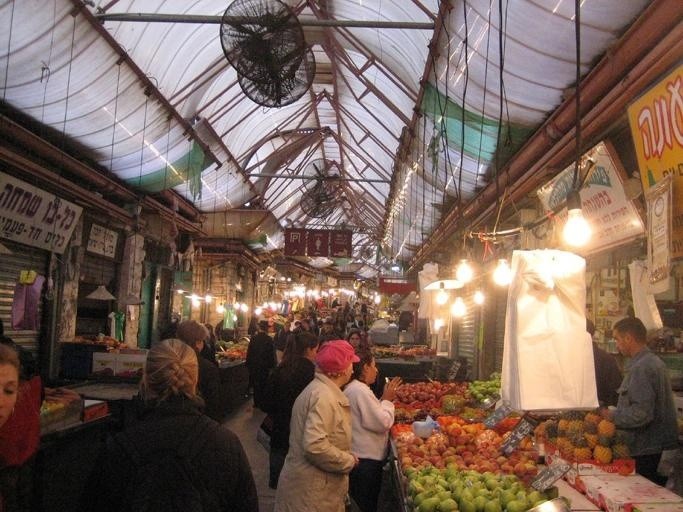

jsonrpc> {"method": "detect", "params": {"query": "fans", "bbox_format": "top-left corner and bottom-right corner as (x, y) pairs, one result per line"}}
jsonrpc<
(220, 2), (304, 80)
(303, 159), (341, 197)
(236, 43), (317, 107)
(299, 186), (336, 219)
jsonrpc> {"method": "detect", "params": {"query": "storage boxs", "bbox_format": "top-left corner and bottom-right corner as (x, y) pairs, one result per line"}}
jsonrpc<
(542, 439), (682, 512)
(91, 347), (149, 379)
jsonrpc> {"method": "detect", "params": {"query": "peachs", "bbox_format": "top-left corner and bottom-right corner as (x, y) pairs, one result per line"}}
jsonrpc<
(397, 415), (538, 487)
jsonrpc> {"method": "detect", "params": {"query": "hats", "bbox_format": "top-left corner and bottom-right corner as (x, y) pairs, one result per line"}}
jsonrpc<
(316, 339), (360, 371)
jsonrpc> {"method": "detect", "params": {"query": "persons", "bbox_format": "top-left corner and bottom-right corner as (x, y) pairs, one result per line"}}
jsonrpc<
(1, 288), (428, 511)
(599, 316), (679, 486)
(585, 317), (622, 405)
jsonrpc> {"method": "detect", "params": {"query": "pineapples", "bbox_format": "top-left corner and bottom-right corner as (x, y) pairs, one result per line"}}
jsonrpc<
(545, 408), (630, 464)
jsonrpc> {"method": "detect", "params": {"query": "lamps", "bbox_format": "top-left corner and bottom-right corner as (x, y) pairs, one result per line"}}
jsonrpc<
(84, 64), (121, 302)
(492, 2), (515, 290)
(423, 0), (485, 333)
(168, 256), (225, 315)
(44, 18), (76, 300)
(119, 96), (145, 306)
(559, 1), (595, 251)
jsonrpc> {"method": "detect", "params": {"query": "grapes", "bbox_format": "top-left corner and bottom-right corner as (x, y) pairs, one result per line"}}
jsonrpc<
(405, 406), (437, 424)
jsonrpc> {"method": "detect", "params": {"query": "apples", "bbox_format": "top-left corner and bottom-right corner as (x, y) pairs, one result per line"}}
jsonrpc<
(393, 379), (469, 404)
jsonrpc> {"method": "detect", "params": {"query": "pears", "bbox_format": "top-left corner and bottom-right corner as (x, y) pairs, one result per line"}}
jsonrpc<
(404, 464), (558, 512)
(468, 374), (501, 404)
